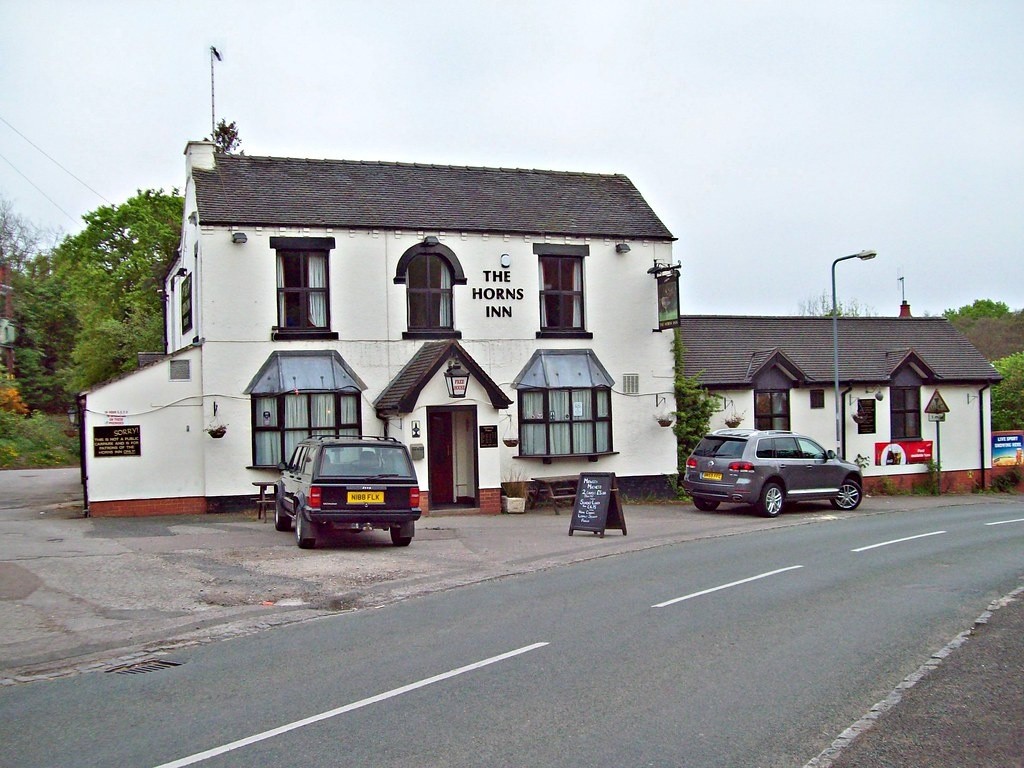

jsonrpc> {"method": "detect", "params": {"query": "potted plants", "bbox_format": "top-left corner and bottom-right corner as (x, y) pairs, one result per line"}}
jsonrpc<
(721, 409), (747, 429)
(653, 410), (675, 427)
(203, 423), (229, 438)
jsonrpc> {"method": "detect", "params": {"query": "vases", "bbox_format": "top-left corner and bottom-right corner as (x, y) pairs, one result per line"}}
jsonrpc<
(503, 440), (519, 447)
(502, 495), (526, 514)
(852, 415), (868, 423)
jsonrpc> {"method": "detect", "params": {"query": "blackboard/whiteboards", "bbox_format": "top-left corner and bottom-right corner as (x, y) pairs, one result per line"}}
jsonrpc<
(571, 472), (613, 529)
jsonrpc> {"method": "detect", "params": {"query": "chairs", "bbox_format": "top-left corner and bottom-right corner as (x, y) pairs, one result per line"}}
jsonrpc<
(360, 450), (379, 472)
(323, 455), (333, 473)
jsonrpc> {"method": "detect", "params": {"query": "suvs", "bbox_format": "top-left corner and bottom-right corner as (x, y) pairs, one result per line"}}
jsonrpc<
(274, 434), (422, 549)
(682, 428), (863, 519)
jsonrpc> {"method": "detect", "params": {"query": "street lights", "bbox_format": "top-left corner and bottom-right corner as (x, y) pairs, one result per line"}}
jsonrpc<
(831, 249), (878, 459)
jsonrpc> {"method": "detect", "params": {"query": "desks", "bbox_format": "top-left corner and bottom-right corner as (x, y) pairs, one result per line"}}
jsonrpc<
(252, 482), (275, 524)
(529, 475), (580, 515)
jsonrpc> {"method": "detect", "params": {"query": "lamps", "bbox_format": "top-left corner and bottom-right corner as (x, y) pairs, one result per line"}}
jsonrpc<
(424, 236), (439, 246)
(866, 384), (884, 401)
(443, 355), (469, 398)
(174, 268), (188, 277)
(232, 233), (247, 243)
(615, 244), (631, 254)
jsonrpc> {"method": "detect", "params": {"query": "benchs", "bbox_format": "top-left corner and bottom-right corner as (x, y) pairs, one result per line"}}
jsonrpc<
(547, 494), (577, 502)
(250, 497), (272, 505)
(526, 488), (575, 497)
(257, 500), (275, 510)
